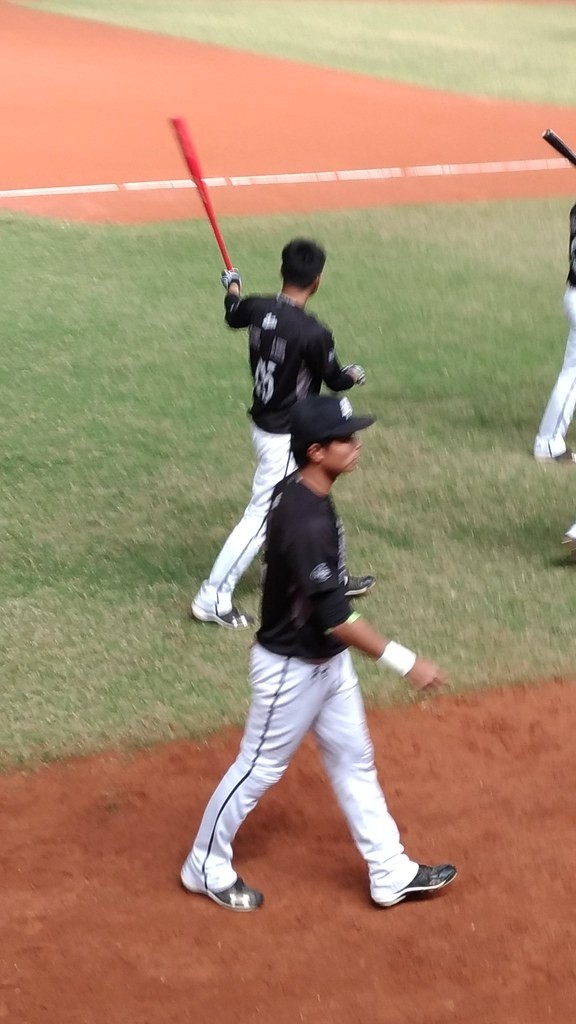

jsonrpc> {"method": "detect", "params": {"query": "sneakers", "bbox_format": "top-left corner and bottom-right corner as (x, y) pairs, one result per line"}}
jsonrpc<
(189, 601), (254, 630)
(374, 864), (457, 907)
(180, 873), (264, 912)
(340, 576), (375, 596)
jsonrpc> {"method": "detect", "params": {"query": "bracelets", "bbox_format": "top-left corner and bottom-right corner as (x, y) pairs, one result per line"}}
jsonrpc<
(375, 641), (416, 679)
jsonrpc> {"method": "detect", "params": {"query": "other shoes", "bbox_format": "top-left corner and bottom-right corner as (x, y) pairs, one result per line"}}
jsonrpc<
(534, 451), (576, 464)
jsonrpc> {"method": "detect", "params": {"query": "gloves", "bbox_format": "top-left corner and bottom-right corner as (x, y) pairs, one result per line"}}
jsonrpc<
(221, 267), (242, 291)
(345, 364), (365, 385)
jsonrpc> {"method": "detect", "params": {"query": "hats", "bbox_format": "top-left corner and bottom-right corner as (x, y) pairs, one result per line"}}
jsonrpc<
(288, 396), (376, 442)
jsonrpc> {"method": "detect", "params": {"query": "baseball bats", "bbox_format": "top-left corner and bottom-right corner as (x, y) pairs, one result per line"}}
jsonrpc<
(170, 114), (233, 271)
(541, 126), (576, 166)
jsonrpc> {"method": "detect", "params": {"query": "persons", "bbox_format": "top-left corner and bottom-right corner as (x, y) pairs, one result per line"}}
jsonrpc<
(180, 396), (459, 913)
(533, 202), (576, 463)
(189, 237), (377, 630)
